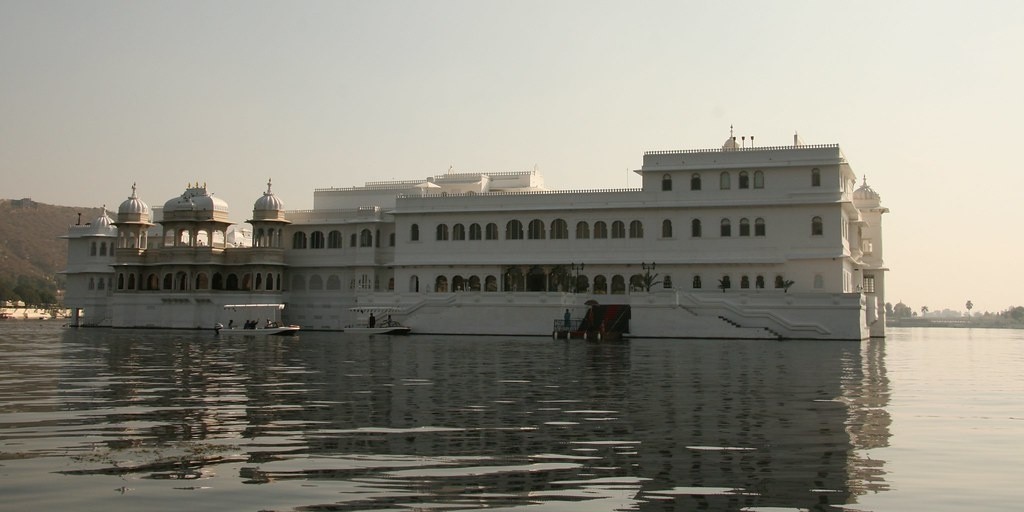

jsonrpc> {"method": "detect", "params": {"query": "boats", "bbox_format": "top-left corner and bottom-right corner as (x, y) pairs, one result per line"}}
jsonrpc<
(552, 300), (631, 344)
(214, 303), (303, 336)
(342, 305), (415, 337)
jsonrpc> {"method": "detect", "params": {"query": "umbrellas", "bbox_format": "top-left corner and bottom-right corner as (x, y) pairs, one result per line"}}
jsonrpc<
(584, 300), (598, 312)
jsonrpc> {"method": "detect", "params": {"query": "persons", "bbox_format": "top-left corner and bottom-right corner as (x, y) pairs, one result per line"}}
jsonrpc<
(243, 318), (259, 329)
(228, 320), (233, 329)
(588, 308), (594, 330)
(267, 317), (272, 323)
(552, 279), (563, 292)
(388, 315), (394, 327)
(564, 308), (571, 330)
(369, 313), (376, 328)
